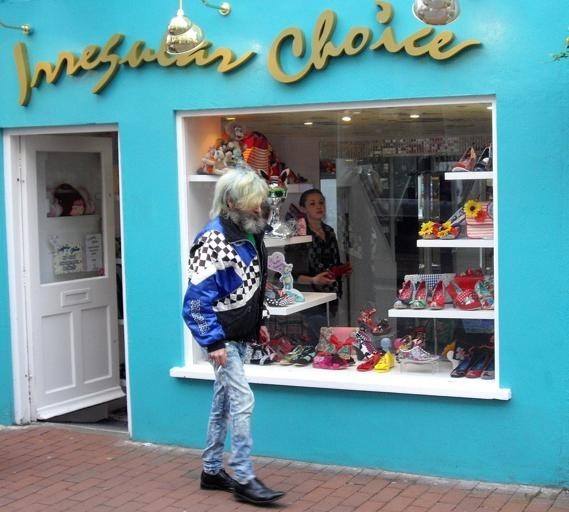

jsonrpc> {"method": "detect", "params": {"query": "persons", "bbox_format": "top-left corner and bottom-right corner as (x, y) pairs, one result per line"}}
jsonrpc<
(283, 189), (354, 346)
(181, 166), (286, 503)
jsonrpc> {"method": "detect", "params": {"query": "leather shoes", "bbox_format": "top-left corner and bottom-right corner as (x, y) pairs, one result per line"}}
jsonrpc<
(199, 468), (239, 493)
(233, 476), (288, 505)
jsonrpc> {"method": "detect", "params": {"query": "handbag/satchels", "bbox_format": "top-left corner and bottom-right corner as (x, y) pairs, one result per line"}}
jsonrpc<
(464, 192), (493, 238)
(316, 327), (359, 354)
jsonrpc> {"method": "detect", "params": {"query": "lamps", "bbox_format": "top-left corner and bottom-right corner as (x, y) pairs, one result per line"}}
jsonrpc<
(165, 0), (231, 55)
(412, 0), (460, 24)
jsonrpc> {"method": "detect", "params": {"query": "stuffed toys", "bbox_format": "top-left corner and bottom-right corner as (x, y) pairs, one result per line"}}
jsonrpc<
(196, 121), (309, 184)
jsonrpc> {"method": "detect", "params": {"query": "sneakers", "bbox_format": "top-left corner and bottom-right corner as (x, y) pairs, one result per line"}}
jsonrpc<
(450, 350), (479, 378)
(398, 346), (439, 364)
(373, 351), (394, 373)
(465, 352), (490, 378)
(480, 353), (495, 380)
(284, 345), (304, 362)
(313, 353), (354, 369)
(297, 346), (316, 363)
(356, 351), (382, 372)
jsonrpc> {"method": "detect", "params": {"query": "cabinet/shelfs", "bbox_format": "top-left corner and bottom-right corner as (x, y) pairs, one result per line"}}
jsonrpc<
(189, 174), (337, 316)
(387, 171), (495, 321)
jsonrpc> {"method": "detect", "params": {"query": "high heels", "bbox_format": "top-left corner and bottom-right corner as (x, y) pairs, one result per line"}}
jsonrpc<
(451, 146), (477, 172)
(447, 280), (481, 310)
(472, 147), (492, 171)
(474, 280), (494, 310)
(265, 285), (287, 307)
(266, 281), (295, 303)
(428, 280), (446, 310)
(411, 281), (427, 309)
(393, 280), (414, 309)
(357, 308), (391, 335)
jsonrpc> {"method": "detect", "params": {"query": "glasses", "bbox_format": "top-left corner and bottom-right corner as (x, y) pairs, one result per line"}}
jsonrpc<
(266, 187), (285, 198)
